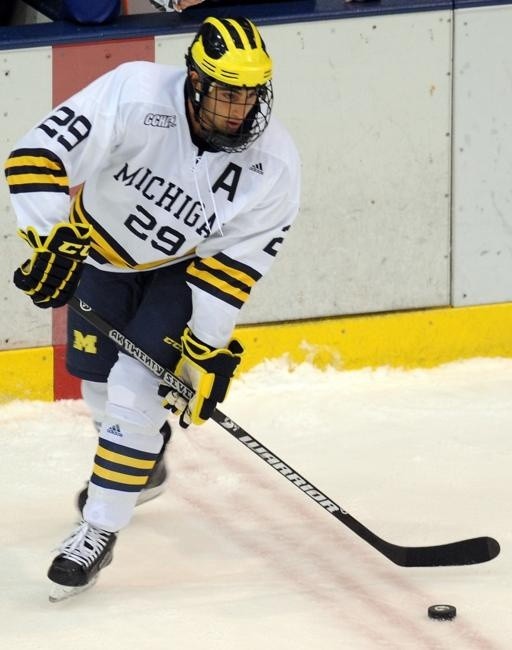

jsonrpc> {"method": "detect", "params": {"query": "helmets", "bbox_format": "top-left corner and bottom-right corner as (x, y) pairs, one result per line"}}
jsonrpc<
(188, 16), (275, 87)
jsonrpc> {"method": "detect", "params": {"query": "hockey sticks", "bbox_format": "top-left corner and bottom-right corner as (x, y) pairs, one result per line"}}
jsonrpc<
(67, 295), (500, 567)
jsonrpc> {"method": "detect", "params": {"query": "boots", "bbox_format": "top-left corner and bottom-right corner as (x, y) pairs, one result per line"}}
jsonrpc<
(44, 525), (118, 589)
(77, 418), (174, 516)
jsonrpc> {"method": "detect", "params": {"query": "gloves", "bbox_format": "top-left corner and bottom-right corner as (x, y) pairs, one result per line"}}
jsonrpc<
(155, 324), (245, 431)
(14, 221), (88, 311)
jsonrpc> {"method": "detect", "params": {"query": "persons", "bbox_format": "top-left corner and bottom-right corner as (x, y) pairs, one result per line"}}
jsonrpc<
(1, 11), (306, 591)
